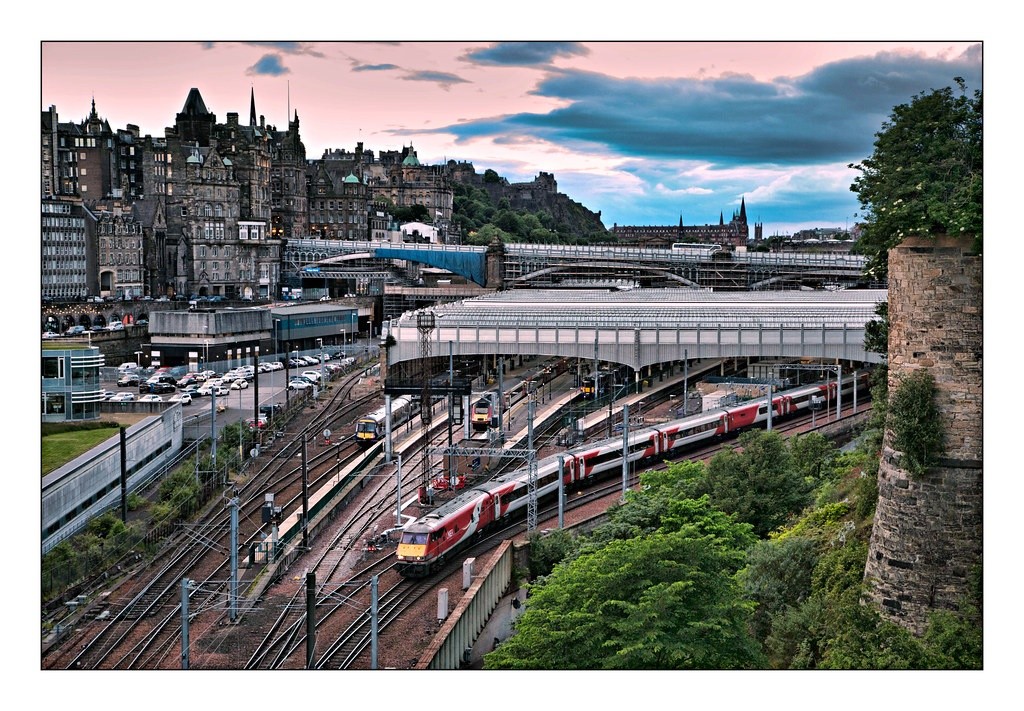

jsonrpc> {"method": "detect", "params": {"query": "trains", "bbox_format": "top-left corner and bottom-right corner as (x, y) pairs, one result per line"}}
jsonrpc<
(395, 372), (867, 580)
(471, 358), (573, 432)
(356, 368), (452, 446)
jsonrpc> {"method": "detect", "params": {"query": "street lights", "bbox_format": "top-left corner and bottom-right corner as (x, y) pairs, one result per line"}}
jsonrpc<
(366, 320), (372, 363)
(274, 318), (279, 362)
(292, 350), (299, 406)
(235, 379), (242, 461)
(202, 325), (208, 377)
(340, 328), (346, 390)
(350, 312), (356, 354)
(319, 337), (326, 394)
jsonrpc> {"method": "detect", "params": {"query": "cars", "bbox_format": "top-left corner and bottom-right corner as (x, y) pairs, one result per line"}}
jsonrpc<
(85, 294), (228, 304)
(40, 319), (147, 339)
(288, 352), (361, 391)
(117, 352), (286, 396)
(243, 403), (284, 431)
(104, 389), (189, 405)
